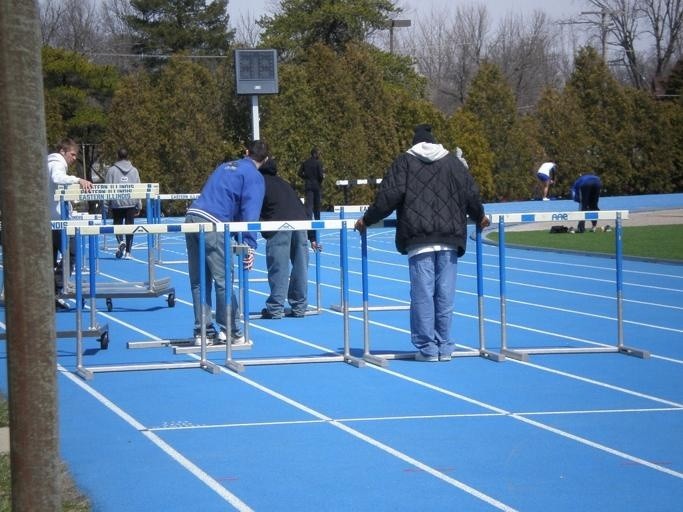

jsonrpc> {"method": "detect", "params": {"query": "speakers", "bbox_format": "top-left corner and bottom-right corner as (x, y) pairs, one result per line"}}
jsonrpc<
(233, 49), (279, 95)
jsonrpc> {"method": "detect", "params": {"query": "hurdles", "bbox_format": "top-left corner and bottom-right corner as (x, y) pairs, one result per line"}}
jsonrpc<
(0, 178), (649, 380)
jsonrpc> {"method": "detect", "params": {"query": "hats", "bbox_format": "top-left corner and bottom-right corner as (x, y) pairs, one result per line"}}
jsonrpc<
(413, 124), (436, 145)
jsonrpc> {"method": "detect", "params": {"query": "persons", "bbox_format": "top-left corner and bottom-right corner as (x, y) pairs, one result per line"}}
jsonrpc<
(537, 162), (557, 201)
(297, 148), (323, 220)
(103, 148), (142, 260)
(259, 161), (322, 319)
(185, 140), (269, 345)
(571, 175), (601, 232)
(354, 125), (491, 361)
(47, 137), (94, 308)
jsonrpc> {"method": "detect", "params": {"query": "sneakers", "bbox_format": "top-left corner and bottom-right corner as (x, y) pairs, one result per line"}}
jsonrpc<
(116, 241), (135, 259)
(415, 351), (452, 362)
(194, 331), (253, 345)
